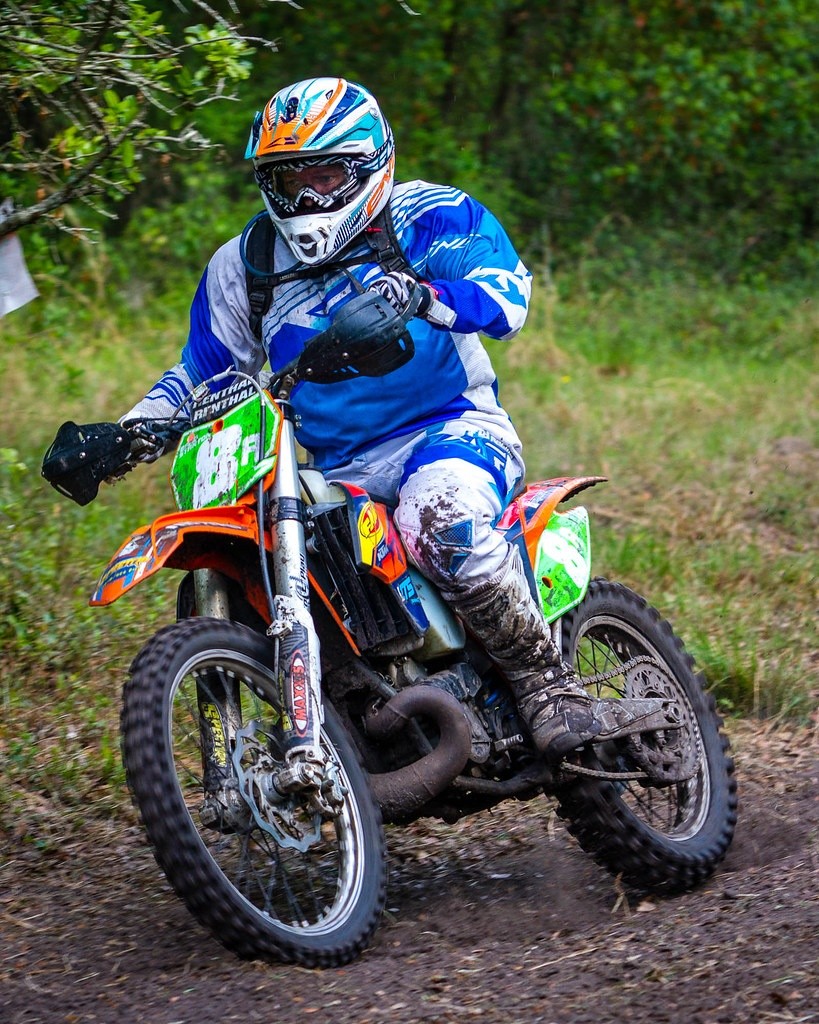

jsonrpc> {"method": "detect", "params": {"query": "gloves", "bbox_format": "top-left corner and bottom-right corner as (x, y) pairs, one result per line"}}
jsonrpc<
(364, 269), (428, 318)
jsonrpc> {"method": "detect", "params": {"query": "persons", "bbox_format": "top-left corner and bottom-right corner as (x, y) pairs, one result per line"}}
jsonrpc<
(81, 76), (601, 755)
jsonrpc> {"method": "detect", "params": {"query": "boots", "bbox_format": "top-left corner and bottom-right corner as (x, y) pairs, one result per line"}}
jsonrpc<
(441, 541), (600, 753)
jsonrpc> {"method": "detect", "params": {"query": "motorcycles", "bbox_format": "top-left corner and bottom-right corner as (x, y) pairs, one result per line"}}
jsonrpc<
(40, 285), (741, 968)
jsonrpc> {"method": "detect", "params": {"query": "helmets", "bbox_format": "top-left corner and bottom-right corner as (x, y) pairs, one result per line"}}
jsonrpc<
(244, 77), (395, 266)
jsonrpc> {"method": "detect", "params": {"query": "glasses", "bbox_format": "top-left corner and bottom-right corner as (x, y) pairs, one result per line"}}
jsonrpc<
(259, 155), (359, 213)
